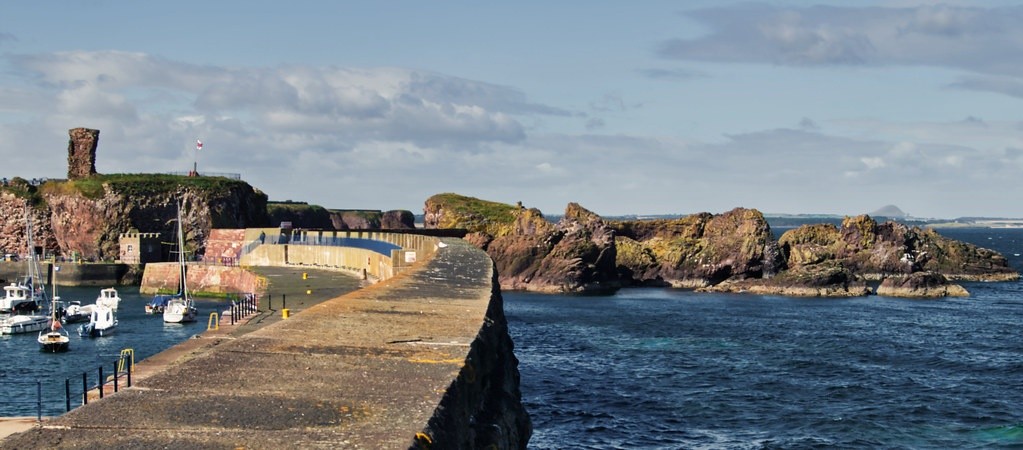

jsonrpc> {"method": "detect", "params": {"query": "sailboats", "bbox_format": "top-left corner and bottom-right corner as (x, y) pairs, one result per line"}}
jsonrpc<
(0, 203), (99, 335)
(162, 200), (199, 323)
(37, 254), (71, 353)
(145, 188), (192, 314)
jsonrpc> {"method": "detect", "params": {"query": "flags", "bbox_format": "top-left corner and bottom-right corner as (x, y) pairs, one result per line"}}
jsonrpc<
(197, 139), (202, 150)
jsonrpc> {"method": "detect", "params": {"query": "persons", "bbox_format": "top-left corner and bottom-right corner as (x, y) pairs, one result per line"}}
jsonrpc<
(260, 230), (266, 244)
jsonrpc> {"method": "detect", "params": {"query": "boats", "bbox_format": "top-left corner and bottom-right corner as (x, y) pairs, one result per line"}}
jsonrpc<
(76, 296), (119, 337)
(96, 287), (122, 313)
